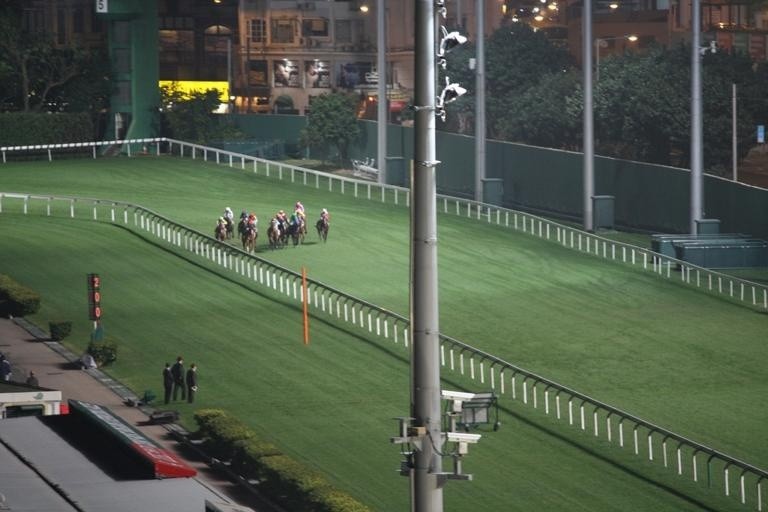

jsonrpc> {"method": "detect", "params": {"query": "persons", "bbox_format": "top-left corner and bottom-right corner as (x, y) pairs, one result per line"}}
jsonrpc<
(0, 354), (12, 381)
(215, 201), (330, 235)
(27, 370), (40, 387)
(171, 357), (186, 400)
(186, 363), (198, 404)
(162, 362), (174, 404)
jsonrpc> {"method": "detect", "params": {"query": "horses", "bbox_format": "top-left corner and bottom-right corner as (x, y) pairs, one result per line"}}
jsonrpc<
(265, 212), (307, 251)
(313, 216), (330, 246)
(212, 215), (259, 254)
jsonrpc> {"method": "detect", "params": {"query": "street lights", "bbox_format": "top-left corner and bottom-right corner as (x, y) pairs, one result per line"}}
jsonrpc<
(596, 34), (639, 82)
